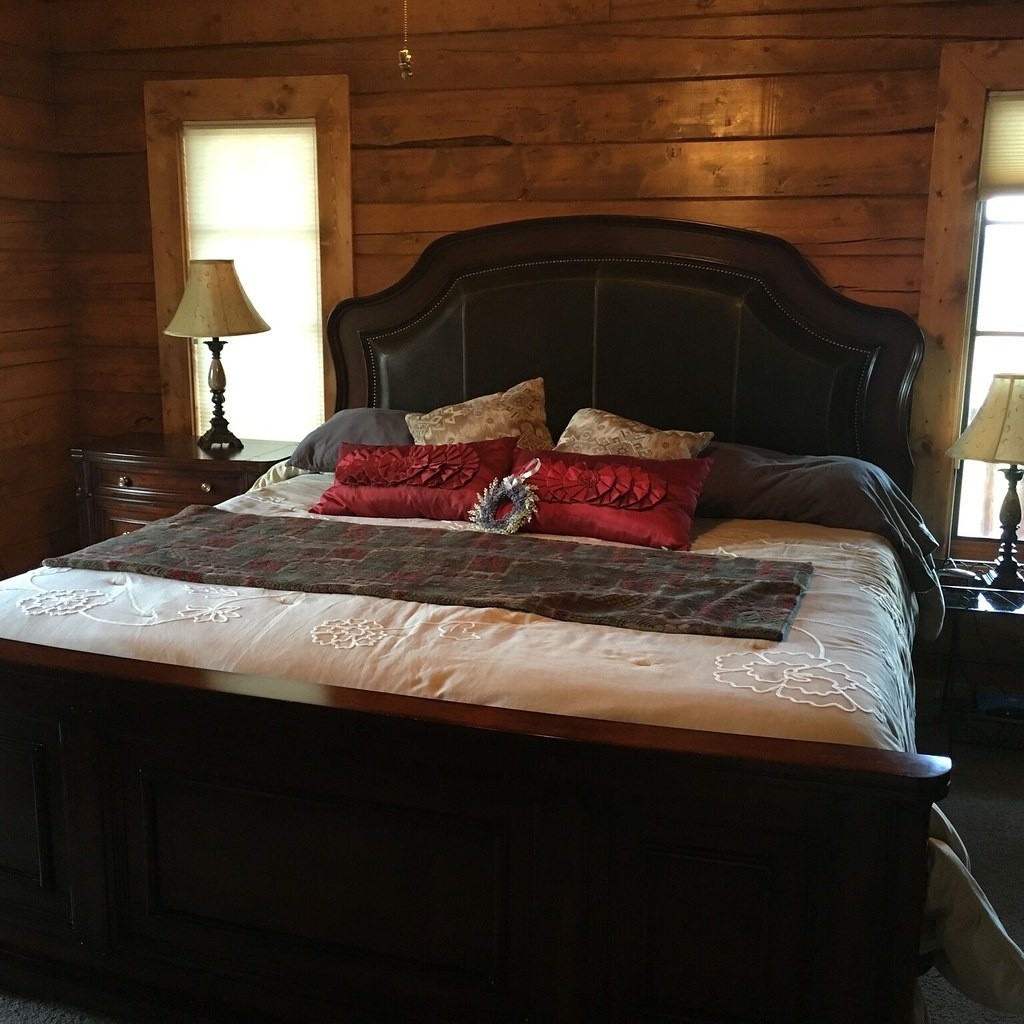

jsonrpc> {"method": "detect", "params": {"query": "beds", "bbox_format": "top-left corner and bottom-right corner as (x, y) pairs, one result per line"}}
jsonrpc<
(0, 210), (1024, 1024)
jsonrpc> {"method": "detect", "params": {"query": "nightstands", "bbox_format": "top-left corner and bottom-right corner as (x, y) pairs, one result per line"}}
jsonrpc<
(71, 430), (302, 554)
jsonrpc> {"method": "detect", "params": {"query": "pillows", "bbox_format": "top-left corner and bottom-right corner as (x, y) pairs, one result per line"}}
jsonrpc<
(309, 434), (522, 524)
(405, 378), (554, 452)
(284, 407), (416, 472)
(695, 440), (939, 593)
(551, 408), (714, 461)
(496, 446), (713, 552)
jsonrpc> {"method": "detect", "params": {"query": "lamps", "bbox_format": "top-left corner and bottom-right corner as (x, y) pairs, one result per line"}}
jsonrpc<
(163, 260), (271, 452)
(944, 373), (1024, 591)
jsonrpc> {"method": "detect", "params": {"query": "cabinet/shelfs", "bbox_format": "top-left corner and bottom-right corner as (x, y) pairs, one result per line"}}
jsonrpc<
(927, 557), (1024, 748)
